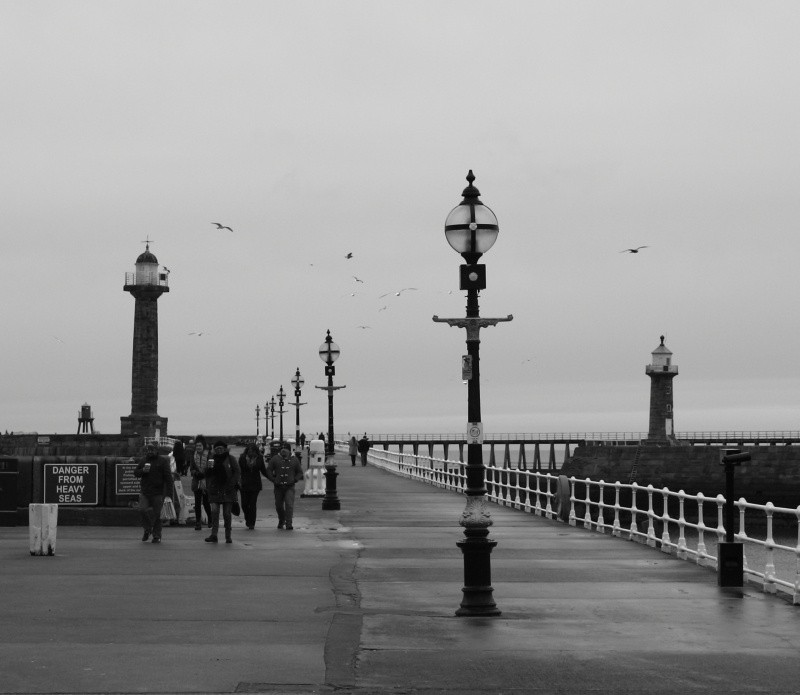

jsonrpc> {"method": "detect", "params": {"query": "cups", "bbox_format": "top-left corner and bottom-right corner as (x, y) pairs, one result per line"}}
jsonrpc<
(142, 463), (151, 473)
(207, 458), (214, 467)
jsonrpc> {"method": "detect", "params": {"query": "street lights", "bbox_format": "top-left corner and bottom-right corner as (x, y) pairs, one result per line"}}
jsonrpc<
(270, 396), (275, 439)
(277, 384), (285, 444)
(291, 368), (306, 466)
(319, 330), (342, 509)
(255, 404), (260, 435)
(264, 401), (270, 439)
(445, 169), (509, 616)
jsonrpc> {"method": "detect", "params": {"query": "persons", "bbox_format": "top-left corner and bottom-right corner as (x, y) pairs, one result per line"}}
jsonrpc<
(267, 444), (304, 531)
(348, 437), (359, 467)
(357, 436), (370, 467)
(133, 440), (174, 542)
(319, 433), (325, 441)
(173, 439), (195, 476)
(190, 435), (213, 530)
(203, 441), (241, 543)
(237, 443), (276, 529)
(300, 433), (306, 450)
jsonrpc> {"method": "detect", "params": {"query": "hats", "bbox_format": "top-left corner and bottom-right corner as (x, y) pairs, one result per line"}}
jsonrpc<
(280, 442), (291, 451)
(214, 440), (228, 448)
(146, 440), (159, 450)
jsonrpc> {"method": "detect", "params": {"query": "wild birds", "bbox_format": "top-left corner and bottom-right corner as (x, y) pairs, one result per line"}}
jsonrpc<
(344, 252), (352, 259)
(353, 275), (364, 285)
(378, 287), (417, 298)
(350, 292), (387, 329)
(187, 332), (202, 337)
(447, 289), (455, 295)
(618, 244), (650, 253)
(211, 222), (233, 232)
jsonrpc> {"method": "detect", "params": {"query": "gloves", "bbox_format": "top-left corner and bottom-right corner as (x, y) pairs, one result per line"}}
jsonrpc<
(198, 473), (203, 479)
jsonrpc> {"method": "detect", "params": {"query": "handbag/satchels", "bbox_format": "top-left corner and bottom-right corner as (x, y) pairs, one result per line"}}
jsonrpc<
(159, 496), (176, 520)
(232, 501), (240, 515)
(198, 479), (207, 491)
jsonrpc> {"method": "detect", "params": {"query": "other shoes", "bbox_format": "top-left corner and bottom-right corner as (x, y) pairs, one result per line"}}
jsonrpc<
(226, 538), (233, 544)
(142, 531), (151, 541)
(195, 524), (201, 530)
(152, 537), (161, 543)
(205, 536), (218, 542)
